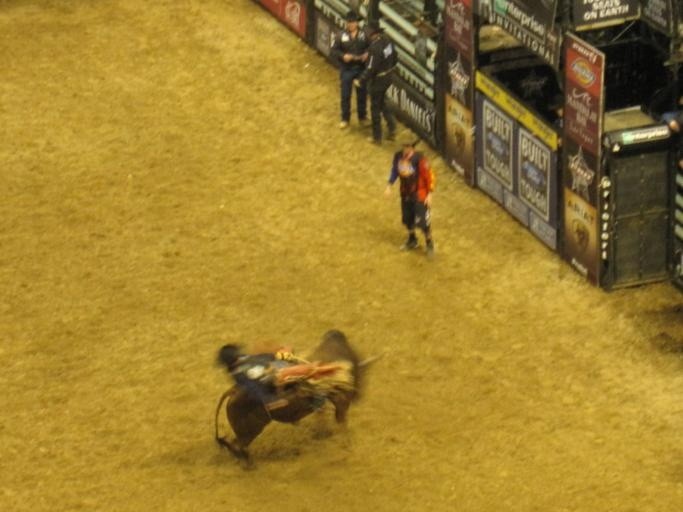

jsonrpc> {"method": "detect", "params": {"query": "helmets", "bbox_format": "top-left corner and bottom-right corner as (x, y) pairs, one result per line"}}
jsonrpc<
(221, 345), (239, 364)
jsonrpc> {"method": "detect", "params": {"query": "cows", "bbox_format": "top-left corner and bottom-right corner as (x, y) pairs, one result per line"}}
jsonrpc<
(213, 329), (387, 471)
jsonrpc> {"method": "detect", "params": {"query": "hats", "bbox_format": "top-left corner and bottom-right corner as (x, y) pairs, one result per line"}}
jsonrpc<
(343, 10), (363, 22)
(366, 22), (384, 37)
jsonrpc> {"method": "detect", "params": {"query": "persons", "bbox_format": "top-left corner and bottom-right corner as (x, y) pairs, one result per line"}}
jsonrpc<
(382, 129), (437, 258)
(332, 8), (372, 130)
(353, 23), (398, 145)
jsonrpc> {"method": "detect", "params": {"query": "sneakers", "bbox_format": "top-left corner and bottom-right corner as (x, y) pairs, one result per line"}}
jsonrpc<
(394, 128), (419, 146)
(427, 239), (434, 257)
(399, 238), (418, 251)
(338, 117), (393, 144)
